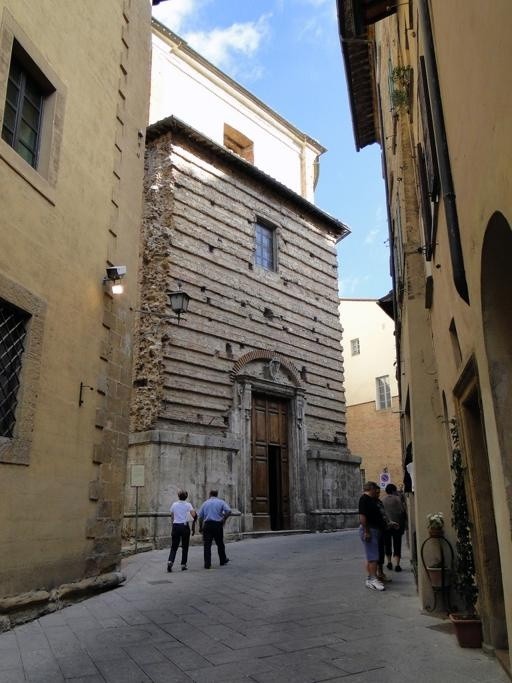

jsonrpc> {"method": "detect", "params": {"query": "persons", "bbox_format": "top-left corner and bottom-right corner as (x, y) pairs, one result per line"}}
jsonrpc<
(397, 483), (402, 496)
(358, 481), (387, 593)
(380, 483), (409, 572)
(374, 485), (400, 581)
(167, 490), (198, 570)
(198, 490), (232, 569)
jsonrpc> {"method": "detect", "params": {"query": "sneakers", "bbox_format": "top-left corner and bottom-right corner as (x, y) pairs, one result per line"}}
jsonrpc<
(387, 564), (392, 569)
(396, 566), (402, 571)
(368, 578), (385, 590)
(365, 579), (384, 586)
(379, 573), (392, 582)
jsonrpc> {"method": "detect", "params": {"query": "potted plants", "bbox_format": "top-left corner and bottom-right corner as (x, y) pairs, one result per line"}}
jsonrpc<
(426, 512), (483, 649)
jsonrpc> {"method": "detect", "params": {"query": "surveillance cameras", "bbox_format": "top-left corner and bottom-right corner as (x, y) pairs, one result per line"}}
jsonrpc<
(107, 265), (128, 278)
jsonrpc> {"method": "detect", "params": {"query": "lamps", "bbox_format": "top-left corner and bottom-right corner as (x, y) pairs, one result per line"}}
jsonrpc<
(129, 292), (193, 325)
(103, 279), (127, 294)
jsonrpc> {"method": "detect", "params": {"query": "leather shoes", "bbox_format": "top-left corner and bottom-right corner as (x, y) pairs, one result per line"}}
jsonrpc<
(181, 565), (188, 569)
